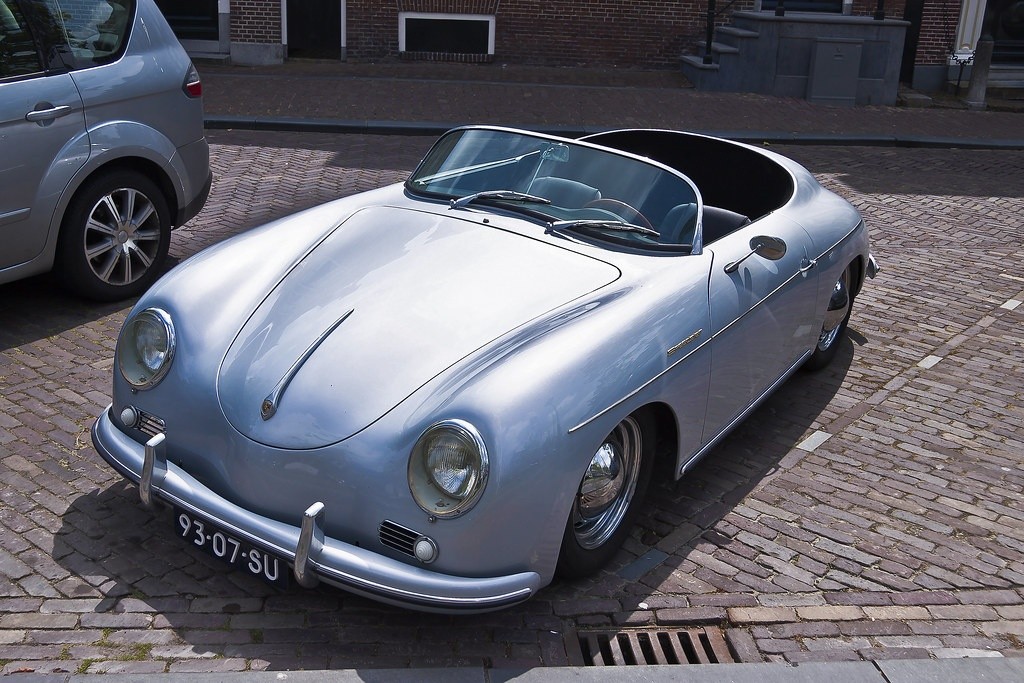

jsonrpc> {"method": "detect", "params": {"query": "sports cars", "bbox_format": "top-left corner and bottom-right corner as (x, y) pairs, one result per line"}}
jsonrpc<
(91, 123), (881, 613)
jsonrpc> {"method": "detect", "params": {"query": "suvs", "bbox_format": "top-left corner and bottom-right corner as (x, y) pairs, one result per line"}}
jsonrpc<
(0, 0), (213, 302)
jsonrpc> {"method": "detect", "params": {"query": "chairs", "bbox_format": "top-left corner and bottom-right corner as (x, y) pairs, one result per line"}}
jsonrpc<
(656, 202), (750, 246)
(526, 175), (601, 211)
